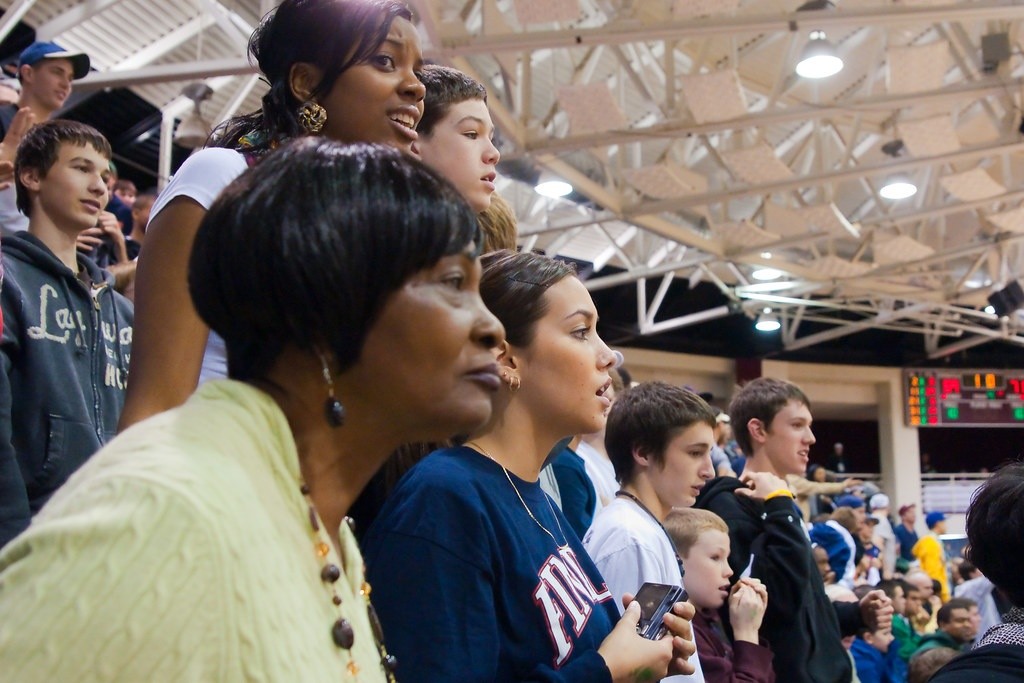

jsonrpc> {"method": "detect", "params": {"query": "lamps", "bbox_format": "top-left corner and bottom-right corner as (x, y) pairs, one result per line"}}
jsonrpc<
(173, 6), (213, 149)
(534, 167), (573, 199)
(754, 305), (780, 332)
(794, 30), (843, 80)
(877, 171), (917, 199)
(987, 281), (1024, 320)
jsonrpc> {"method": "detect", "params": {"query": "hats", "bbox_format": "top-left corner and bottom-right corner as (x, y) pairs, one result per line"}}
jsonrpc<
(17, 42), (89, 80)
(869, 494), (889, 508)
(898, 502), (916, 517)
(837, 495), (865, 510)
(926, 512), (948, 529)
(864, 512), (881, 524)
(715, 411), (730, 424)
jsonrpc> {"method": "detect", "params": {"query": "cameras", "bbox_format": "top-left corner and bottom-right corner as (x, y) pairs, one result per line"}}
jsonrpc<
(630, 583), (689, 642)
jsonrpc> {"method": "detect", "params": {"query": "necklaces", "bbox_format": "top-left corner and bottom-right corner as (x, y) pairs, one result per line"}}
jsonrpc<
(300, 474), (397, 683)
(615, 491), (686, 577)
(466, 440), (569, 552)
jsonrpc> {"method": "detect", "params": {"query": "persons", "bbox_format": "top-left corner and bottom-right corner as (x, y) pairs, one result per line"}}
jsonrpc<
(0, 0), (1024, 683)
